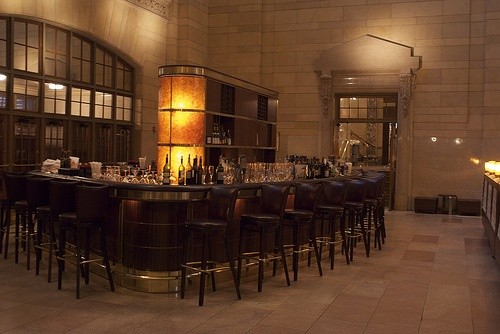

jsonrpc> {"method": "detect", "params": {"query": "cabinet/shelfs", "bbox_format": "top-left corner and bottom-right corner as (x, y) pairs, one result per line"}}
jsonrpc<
(157, 108), (278, 149)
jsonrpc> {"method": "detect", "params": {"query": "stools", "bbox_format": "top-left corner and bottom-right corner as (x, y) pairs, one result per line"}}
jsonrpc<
(0, 171), (386, 307)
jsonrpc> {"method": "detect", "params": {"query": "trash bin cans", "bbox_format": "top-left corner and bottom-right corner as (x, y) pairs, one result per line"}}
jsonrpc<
(444, 195), (457, 215)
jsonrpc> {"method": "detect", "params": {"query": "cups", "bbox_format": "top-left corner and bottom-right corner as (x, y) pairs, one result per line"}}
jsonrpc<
(244, 161), (294, 183)
(90, 162), (101, 180)
(70, 157), (79, 168)
(139, 158), (146, 170)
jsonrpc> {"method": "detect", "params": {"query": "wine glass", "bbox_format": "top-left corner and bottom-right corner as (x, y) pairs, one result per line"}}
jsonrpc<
(103, 165), (162, 185)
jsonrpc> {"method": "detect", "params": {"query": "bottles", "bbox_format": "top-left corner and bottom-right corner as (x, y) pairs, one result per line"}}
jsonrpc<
(206, 123), (232, 145)
(162, 154), (170, 184)
(284, 155), (352, 178)
(178, 154), (244, 185)
